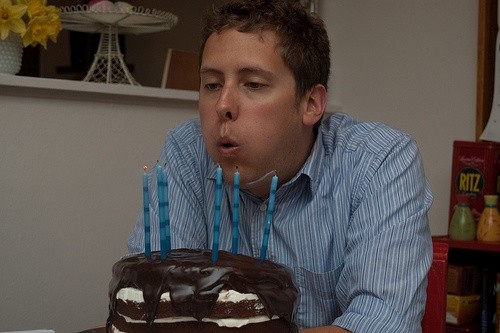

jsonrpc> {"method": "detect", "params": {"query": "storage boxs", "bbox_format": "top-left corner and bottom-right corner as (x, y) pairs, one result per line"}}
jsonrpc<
(444, 263), (481, 326)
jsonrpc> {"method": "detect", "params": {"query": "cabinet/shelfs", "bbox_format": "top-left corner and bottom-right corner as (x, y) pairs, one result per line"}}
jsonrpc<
(416, 235), (500, 333)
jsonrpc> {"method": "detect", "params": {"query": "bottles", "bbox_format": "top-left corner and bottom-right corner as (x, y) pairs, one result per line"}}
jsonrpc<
(449, 195), (476, 241)
(476, 195), (500, 242)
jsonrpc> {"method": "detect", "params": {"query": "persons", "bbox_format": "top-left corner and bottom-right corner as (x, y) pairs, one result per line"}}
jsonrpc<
(127, 0), (435, 333)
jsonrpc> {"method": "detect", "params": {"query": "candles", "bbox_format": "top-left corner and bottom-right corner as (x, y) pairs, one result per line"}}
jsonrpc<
(232, 165), (240, 256)
(260, 171), (279, 262)
(211, 161), (222, 265)
(142, 160), (171, 260)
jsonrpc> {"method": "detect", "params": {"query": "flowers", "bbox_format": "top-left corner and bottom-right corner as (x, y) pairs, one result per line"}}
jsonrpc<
(0, 0), (65, 50)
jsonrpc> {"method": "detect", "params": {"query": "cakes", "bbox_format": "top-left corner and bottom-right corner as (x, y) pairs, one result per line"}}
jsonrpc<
(105, 248), (301, 333)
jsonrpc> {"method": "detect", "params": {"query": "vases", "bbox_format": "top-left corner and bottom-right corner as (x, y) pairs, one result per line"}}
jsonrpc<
(0, 30), (25, 76)
